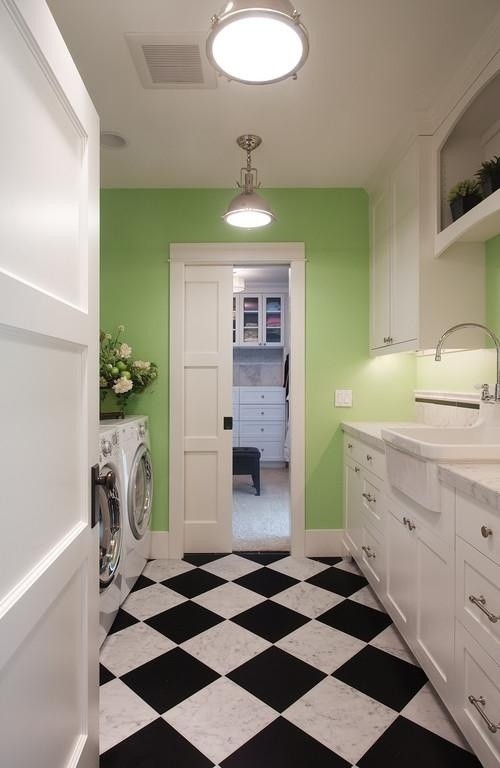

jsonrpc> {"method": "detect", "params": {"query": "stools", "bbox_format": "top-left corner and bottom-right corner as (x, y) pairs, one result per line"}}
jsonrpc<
(233, 447), (261, 495)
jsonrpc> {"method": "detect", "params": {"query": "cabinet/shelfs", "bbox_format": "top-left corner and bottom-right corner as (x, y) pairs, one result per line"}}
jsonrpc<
(370, 134), (486, 362)
(239, 292), (288, 349)
(340, 430), (500, 768)
(233, 292), (240, 348)
(233, 385), (288, 469)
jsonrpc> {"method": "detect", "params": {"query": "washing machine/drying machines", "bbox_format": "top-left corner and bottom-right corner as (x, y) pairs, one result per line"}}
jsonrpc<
(99, 425), (124, 650)
(100, 415), (155, 606)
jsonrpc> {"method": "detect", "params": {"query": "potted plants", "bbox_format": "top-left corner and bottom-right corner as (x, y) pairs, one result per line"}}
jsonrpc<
(475, 154), (499, 195)
(447, 180), (481, 222)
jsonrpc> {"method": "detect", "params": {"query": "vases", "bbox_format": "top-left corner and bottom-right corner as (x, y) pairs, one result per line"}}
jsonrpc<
(116, 395), (128, 419)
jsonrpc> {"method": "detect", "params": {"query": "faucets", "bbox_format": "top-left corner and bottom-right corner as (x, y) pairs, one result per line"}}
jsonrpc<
(435, 322), (500, 404)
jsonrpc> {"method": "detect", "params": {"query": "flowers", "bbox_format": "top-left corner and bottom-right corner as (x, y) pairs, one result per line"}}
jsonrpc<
(100, 322), (158, 409)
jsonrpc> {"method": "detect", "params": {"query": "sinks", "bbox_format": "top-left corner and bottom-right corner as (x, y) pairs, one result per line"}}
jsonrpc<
(380, 422), (500, 512)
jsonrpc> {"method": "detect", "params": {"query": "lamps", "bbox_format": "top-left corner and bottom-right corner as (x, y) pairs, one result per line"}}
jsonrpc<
(222, 132), (277, 227)
(204, 0), (311, 85)
